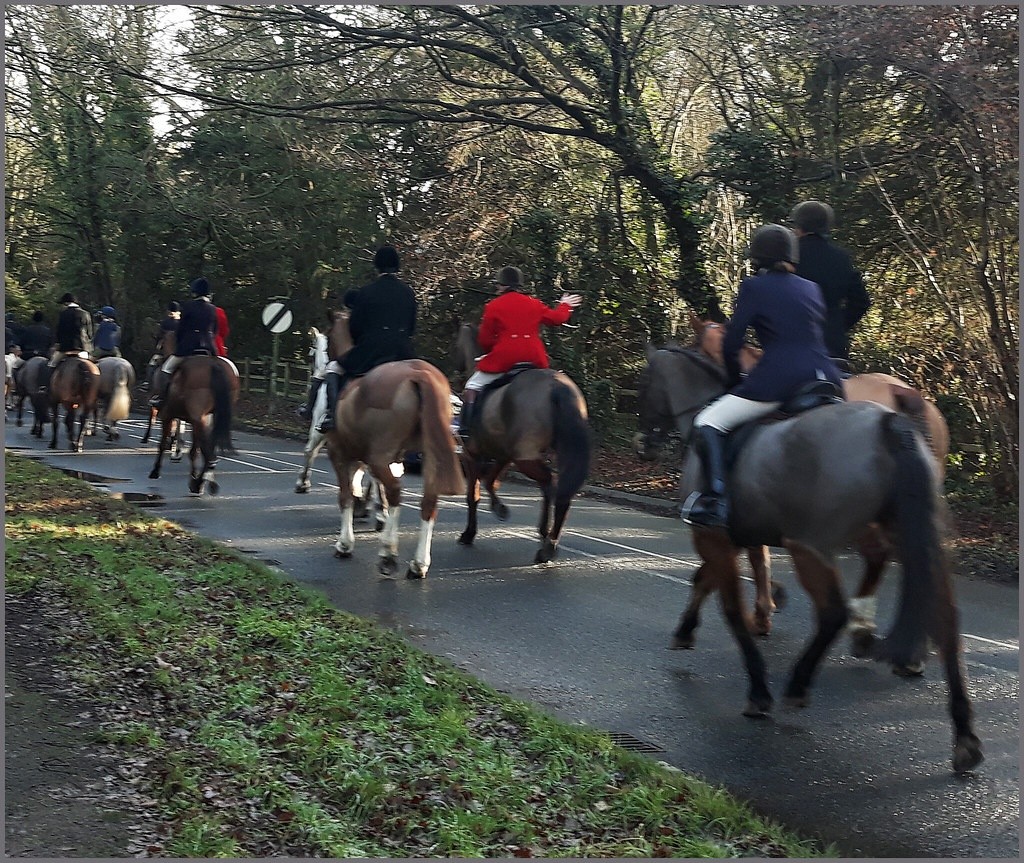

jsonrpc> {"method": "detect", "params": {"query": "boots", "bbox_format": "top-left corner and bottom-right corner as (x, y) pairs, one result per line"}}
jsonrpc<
(458, 389), (477, 435)
(148, 370), (172, 406)
(139, 365), (157, 392)
(298, 378), (322, 420)
(318, 374), (342, 433)
(39, 366), (54, 390)
(678, 427), (728, 526)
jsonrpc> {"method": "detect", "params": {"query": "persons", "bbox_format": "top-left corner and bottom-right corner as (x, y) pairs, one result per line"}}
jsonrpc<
(678, 224), (848, 529)
(785, 200), (870, 359)
(453, 266), (584, 444)
(138, 277), (230, 407)
(5, 293), (122, 411)
(298, 246), (417, 432)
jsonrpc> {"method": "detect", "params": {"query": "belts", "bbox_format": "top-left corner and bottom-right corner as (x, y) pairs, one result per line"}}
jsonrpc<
(100, 347), (111, 351)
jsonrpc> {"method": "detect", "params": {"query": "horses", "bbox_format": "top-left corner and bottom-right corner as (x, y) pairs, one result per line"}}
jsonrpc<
(140, 331), (240, 496)
(92, 357), (136, 441)
(11, 356), (54, 435)
(323, 302), (467, 580)
(635, 337), (988, 777)
(293, 325), (371, 521)
(34, 355), (101, 453)
(688, 310), (950, 677)
(444, 322), (591, 566)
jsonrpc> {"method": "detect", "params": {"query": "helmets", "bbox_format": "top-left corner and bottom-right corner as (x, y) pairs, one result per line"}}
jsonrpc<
(98, 306), (116, 317)
(60, 293), (75, 301)
(750, 223), (794, 261)
(32, 311), (44, 323)
(191, 278), (211, 296)
(167, 301), (182, 312)
(792, 201), (835, 233)
(374, 246), (400, 272)
(493, 267), (525, 287)
